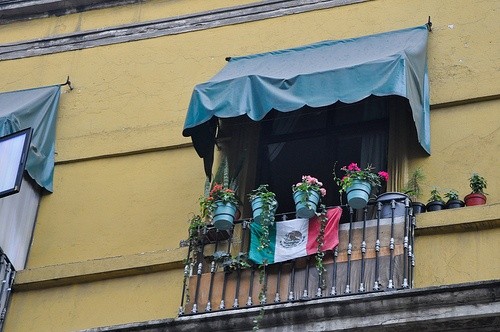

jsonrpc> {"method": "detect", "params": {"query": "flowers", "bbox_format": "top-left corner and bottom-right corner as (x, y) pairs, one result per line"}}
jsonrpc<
(201, 183), (239, 208)
(339, 163), (393, 189)
(291, 175), (330, 199)
(249, 183), (276, 241)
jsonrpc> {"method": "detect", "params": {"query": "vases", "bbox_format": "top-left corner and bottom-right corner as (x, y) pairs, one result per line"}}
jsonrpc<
(344, 180), (372, 209)
(211, 202), (237, 229)
(251, 198), (278, 227)
(293, 189), (322, 218)
(235, 208), (242, 219)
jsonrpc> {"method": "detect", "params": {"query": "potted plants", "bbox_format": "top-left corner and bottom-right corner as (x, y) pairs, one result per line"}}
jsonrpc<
(404, 166), (425, 214)
(425, 184), (446, 212)
(463, 171), (487, 207)
(445, 187), (463, 209)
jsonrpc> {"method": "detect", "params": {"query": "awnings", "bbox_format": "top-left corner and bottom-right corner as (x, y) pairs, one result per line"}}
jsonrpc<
(181, 15), (434, 182)
(0, 77), (77, 193)
